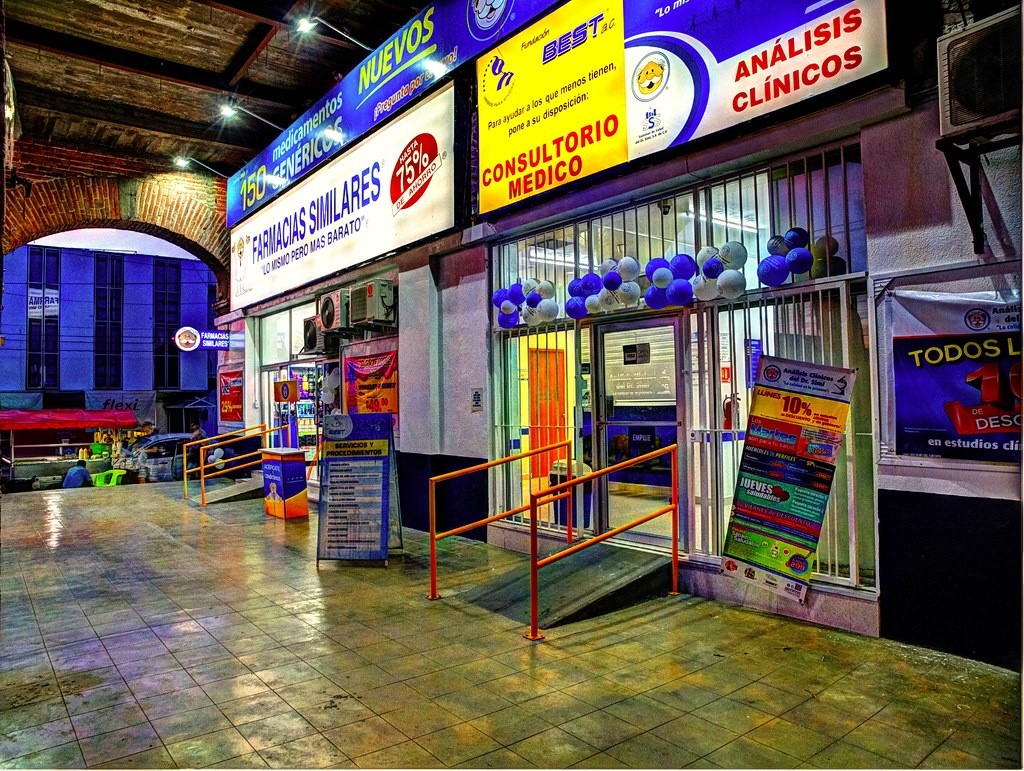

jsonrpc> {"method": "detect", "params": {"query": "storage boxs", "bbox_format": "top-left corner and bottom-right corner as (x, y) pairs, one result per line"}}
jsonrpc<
(90, 443), (112, 455)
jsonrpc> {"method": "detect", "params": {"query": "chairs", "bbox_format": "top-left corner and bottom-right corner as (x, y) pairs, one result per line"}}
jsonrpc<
(95, 469), (127, 488)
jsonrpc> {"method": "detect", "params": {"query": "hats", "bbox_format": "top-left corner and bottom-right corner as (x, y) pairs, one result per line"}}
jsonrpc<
(139, 420), (152, 427)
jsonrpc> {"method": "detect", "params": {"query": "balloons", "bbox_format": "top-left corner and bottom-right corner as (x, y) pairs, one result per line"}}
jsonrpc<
(319, 367), (341, 415)
(493, 227), (844, 330)
(208, 448), (240, 469)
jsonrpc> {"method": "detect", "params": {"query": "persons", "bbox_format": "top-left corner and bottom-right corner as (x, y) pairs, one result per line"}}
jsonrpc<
(267, 482), (282, 501)
(133, 421), (209, 483)
(61, 459), (93, 488)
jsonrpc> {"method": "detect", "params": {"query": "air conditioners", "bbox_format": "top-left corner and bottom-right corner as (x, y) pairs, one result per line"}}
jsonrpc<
(303, 314), (339, 353)
(349, 277), (395, 323)
(320, 288), (350, 332)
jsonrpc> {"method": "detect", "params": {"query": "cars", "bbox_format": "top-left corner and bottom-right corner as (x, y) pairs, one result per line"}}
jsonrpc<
(122, 432), (218, 482)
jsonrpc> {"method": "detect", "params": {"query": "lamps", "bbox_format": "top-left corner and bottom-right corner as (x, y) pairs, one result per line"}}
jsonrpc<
(296, 15), (376, 53)
(173, 156), (229, 180)
(220, 97), (285, 132)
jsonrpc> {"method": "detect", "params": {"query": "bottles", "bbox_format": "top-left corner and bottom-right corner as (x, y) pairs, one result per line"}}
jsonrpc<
(83, 448), (88, 459)
(79, 448), (83, 460)
(88, 448), (92, 459)
